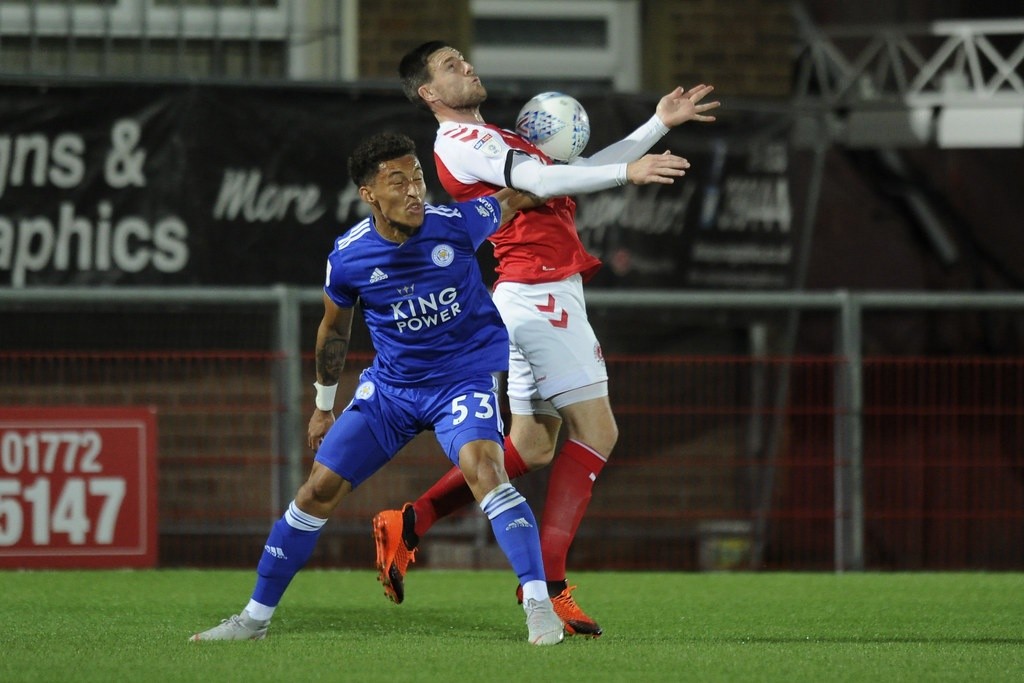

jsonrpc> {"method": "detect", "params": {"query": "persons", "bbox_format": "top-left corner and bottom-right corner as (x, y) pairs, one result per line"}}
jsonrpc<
(189, 130), (565, 647)
(370, 40), (721, 638)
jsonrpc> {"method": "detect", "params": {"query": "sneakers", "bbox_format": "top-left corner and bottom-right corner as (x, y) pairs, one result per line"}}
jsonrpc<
(522, 595), (565, 646)
(515, 577), (603, 638)
(368, 501), (418, 605)
(188, 608), (270, 642)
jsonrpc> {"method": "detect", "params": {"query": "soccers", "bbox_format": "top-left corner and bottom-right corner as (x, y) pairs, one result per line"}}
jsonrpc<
(517, 92), (591, 163)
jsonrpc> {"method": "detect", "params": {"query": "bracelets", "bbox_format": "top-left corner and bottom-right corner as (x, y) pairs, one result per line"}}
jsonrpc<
(312, 382), (339, 412)
